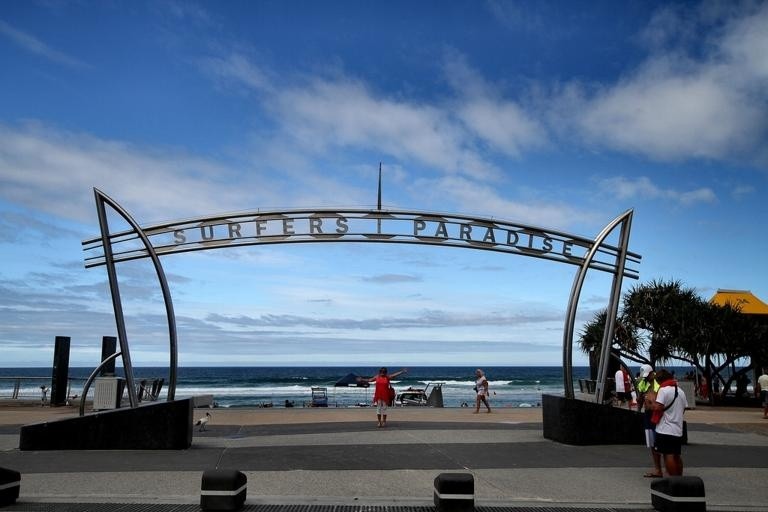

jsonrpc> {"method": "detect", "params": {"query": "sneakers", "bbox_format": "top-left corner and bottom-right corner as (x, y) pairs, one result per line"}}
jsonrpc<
(377, 422), (381, 427)
(381, 423), (385, 427)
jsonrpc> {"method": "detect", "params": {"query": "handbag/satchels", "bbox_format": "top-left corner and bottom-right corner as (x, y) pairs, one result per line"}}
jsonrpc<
(388, 379), (394, 400)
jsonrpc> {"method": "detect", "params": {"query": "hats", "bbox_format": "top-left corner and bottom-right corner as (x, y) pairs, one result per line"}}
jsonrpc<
(639, 364), (652, 378)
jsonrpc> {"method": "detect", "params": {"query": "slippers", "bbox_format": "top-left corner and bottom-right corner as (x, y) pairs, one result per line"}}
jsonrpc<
(643, 472), (662, 478)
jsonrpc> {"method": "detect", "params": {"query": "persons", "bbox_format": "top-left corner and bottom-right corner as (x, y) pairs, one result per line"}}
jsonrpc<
(756, 366), (768, 419)
(643, 369), (688, 476)
(355, 366), (409, 428)
(612, 361), (752, 412)
(635, 362), (668, 478)
(474, 369), (492, 413)
(39, 385), (51, 405)
